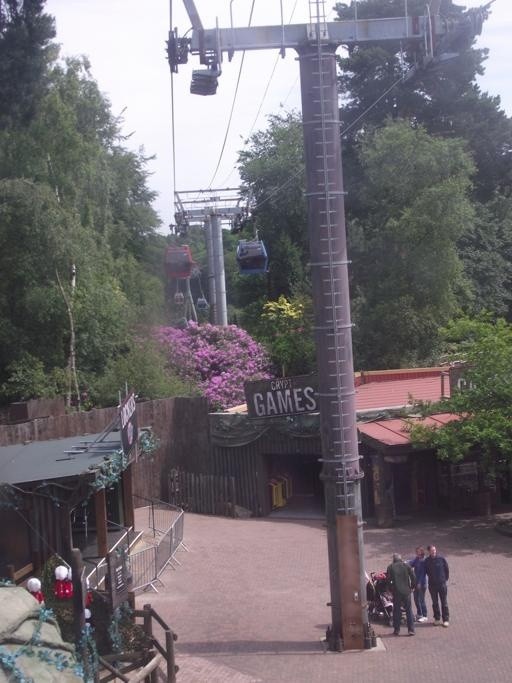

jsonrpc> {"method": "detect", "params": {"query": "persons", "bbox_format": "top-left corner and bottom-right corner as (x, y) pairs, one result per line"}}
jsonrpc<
(409, 545), (428, 622)
(425, 545), (449, 627)
(387, 553), (416, 635)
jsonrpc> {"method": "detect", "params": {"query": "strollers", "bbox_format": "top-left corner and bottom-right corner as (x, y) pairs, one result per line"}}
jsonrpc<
(366, 571), (417, 627)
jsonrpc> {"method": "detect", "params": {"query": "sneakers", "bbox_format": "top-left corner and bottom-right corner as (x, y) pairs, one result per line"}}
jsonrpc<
(415, 614), (428, 622)
(434, 620), (449, 626)
(393, 629), (415, 635)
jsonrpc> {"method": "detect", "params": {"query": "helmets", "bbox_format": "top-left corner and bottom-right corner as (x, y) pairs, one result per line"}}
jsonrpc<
(55, 565), (68, 580)
(27, 578), (42, 592)
